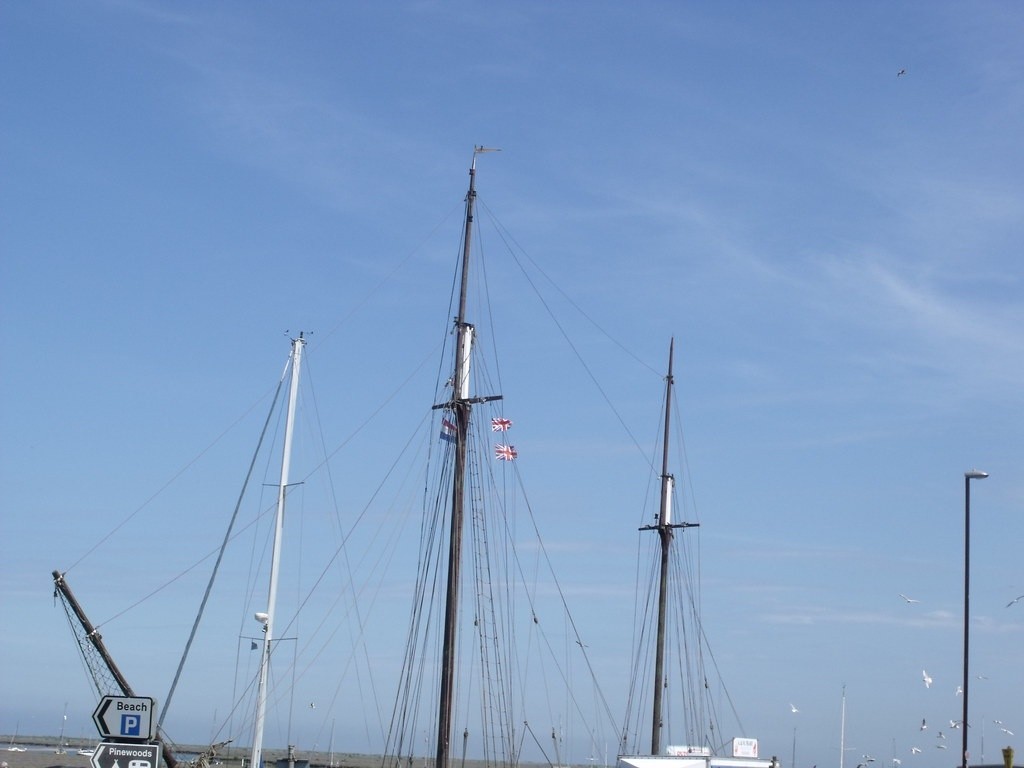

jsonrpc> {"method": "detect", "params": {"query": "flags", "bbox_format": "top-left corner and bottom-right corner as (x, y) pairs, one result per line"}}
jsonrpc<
(495, 444), (517, 462)
(492, 417), (513, 431)
(439, 416), (456, 444)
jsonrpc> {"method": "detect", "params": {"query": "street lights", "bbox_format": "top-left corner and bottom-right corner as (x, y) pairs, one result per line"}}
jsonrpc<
(963, 473), (989, 768)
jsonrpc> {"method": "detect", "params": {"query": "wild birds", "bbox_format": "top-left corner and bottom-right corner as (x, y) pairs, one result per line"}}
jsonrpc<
(899, 593), (920, 606)
(855, 719), (1013, 767)
(789, 703), (799, 713)
(922, 669), (932, 689)
(955, 685), (962, 696)
(1007, 596), (1024, 607)
(307, 702), (316, 709)
